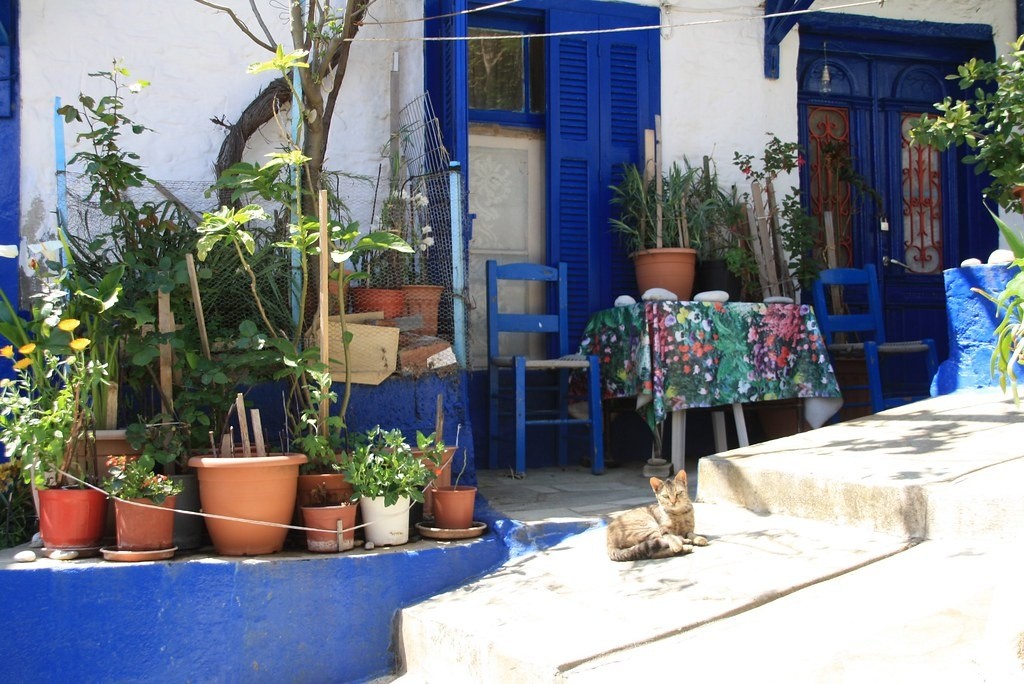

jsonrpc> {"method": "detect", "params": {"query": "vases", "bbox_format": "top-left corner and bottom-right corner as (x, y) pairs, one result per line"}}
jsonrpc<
(351, 287), (404, 322)
(402, 286), (446, 339)
(190, 444), (309, 555)
(743, 358), (870, 444)
(114, 497), (176, 551)
(431, 487), (478, 530)
(383, 448), (457, 519)
(36, 485), (107, 549)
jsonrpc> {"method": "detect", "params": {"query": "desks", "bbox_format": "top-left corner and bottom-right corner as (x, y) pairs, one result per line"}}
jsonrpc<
(569, 303), (842, 476)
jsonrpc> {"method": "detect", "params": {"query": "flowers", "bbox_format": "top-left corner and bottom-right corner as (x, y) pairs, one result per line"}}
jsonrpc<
(97, 451), (185, 504)
(723, 131), (883, 357)
(351, 124), (454, 288)
(0, 319), (109, 489)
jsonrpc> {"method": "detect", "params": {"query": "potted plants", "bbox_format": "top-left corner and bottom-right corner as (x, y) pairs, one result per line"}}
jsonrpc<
(605, 141), (744, 304)
(0, 0), (447, 553)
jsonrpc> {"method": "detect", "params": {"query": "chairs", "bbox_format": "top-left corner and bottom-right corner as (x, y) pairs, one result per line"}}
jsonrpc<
(485, 259), (604, 475)
(812, 261), (939, 425)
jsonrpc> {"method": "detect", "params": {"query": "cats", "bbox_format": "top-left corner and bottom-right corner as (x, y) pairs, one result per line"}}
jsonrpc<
(606, 468), (708, 562)
